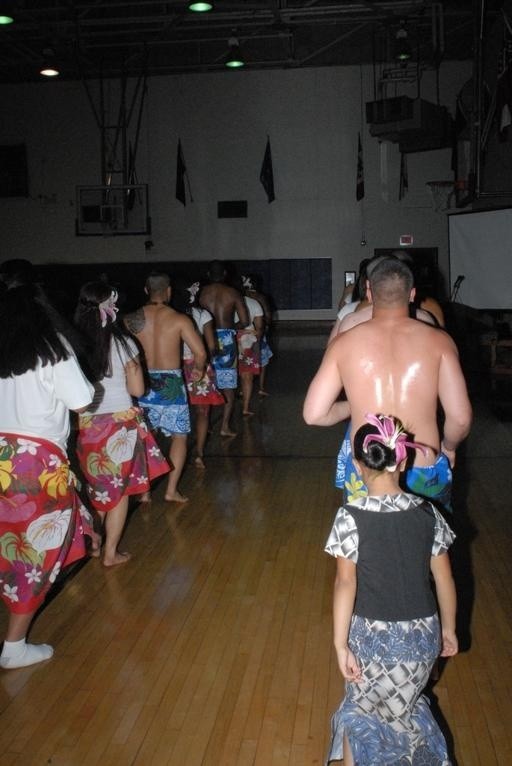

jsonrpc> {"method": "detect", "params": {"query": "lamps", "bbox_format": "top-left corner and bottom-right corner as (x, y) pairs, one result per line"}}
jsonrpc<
(38, 26), (296, 81)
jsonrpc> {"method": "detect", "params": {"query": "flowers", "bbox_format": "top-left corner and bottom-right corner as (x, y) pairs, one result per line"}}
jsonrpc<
(358, 412), (438, 473)
(240, 274), (255, 290)
(97, 287), (121, 330)
(184, 281), (201, 305)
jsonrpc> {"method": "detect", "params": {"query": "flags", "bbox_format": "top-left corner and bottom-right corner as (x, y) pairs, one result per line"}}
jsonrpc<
(356, 131), (364, 201)
(175, 139), (186, 207)
(259, 136), (274, 204)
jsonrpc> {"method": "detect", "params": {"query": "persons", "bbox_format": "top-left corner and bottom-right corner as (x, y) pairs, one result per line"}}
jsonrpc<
(195, 260), (250, 439)
(69, 279), (172, 567)
(237, 272), (275, 397)
(118, 271), (208, 503)
(171, 273), (226, 469)
(0, 259), (97, 671)
(232, 276), (264, 416)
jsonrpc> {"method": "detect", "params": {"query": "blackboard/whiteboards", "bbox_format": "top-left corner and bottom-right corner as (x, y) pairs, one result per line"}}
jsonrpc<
(446, 207), (512, 313)
